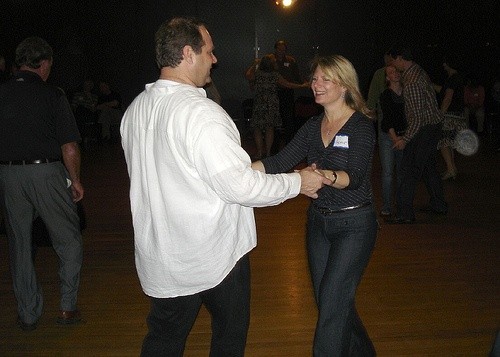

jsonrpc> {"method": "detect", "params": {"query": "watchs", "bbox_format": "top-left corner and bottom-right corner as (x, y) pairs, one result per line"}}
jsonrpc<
(331, 169), (337, 184)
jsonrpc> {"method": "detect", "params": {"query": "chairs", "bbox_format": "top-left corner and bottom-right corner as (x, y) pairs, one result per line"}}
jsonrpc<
(221, 98), (254, 144)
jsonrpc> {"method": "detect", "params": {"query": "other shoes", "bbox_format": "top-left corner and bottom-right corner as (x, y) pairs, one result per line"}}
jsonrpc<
(380, 207), (396, 215)
(419, 203), (448, 215)
(382, 214), (415, 224)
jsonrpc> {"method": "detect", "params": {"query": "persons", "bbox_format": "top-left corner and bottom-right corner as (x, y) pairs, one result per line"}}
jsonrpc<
(245, 39), (311, 160)
(119, 14), (333, 357)
(367, 41), (500, 225)
(251, 53), (378, 357)
(0, 34), (123, 331)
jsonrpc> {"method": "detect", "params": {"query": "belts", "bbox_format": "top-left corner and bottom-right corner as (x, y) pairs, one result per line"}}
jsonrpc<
(311, 200), (371, 215)
(0, 158), (60, 165)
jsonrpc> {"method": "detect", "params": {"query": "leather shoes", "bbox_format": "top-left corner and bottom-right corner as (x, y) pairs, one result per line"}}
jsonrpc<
(15, 314), (37, 330)
(57, 309), (82, 324)
